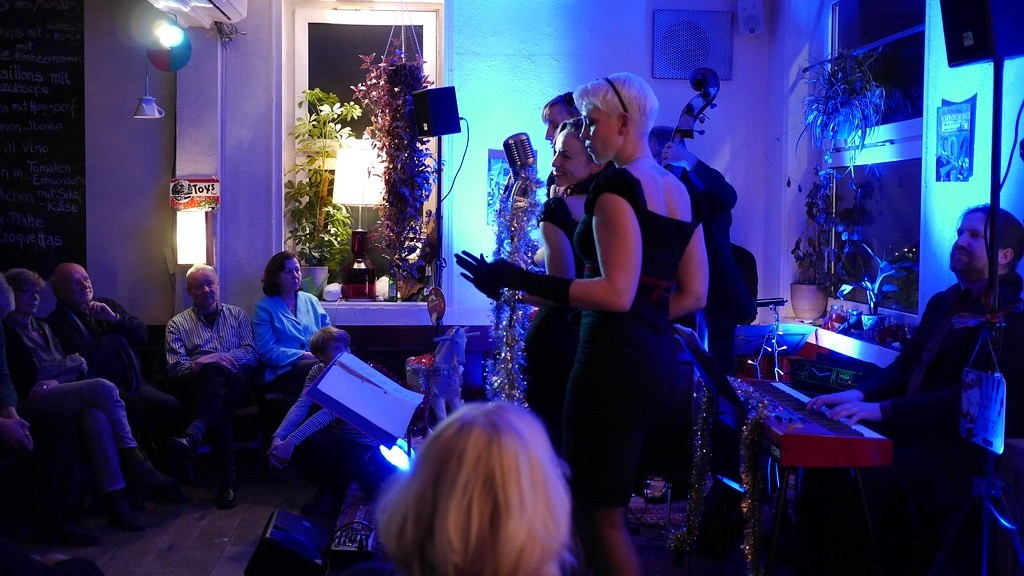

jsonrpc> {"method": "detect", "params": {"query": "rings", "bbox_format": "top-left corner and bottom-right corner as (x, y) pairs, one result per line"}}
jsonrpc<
(43, 386), (46, 389)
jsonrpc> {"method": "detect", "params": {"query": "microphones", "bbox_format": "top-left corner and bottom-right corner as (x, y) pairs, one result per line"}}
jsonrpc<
(503, 133), (536, 218)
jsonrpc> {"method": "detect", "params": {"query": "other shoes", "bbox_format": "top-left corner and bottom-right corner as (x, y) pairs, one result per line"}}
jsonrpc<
(300, 483), (339, 515)
(173, 434), (198, 458)
(216, 488), (237, 509)
(34, 517), (103, 547)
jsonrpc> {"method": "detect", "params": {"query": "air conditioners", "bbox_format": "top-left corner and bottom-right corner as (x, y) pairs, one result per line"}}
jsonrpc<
(183, 0), (248, 26)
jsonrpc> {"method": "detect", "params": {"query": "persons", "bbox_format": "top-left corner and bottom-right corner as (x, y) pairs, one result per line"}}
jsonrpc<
(454, 73), (737, 576)
(0, 251), (410, 576)
(781, 206), (1024, 576)
(374, 400), (572, 576)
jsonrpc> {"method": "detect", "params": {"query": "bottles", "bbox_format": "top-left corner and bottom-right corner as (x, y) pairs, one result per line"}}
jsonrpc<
(829, 305), (845, 319)
(340, 230), (377, 300)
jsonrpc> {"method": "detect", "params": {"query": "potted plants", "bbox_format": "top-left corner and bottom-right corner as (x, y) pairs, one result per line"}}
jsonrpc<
(284, 88), (363, 297)
(772, 49), (912, 340)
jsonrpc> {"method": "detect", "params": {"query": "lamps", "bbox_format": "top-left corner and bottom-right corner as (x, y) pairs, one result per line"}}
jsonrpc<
(134, 1), (160, 118)
(151, 7), (184, 50)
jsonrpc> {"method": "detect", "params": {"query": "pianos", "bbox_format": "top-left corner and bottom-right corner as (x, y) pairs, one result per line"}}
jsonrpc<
(673, 323), (894, 576)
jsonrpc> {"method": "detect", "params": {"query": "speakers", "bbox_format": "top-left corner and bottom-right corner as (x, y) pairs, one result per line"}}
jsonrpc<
(940, 0), (1024, 67)
(244, 507), (333, 576)
(413, 86), (462, 138)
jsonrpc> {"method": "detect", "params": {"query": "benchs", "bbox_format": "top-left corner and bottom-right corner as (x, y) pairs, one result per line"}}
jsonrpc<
(0, 324), (498, 534)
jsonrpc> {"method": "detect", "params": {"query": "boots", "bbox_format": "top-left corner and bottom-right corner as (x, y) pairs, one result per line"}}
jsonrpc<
(105, 489), (144, 532)
(122, 445), (175, 493)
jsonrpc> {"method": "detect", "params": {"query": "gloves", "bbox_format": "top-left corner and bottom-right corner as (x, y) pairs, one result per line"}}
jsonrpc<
(476, 259), (573, 308)
(454, 251), (501, 304)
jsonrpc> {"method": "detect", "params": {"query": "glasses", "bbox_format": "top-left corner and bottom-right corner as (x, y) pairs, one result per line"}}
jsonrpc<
(14, 288), (40, 295)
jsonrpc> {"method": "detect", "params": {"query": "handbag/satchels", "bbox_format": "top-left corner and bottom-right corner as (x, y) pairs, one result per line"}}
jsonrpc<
(958, 329), (1006, 454)
